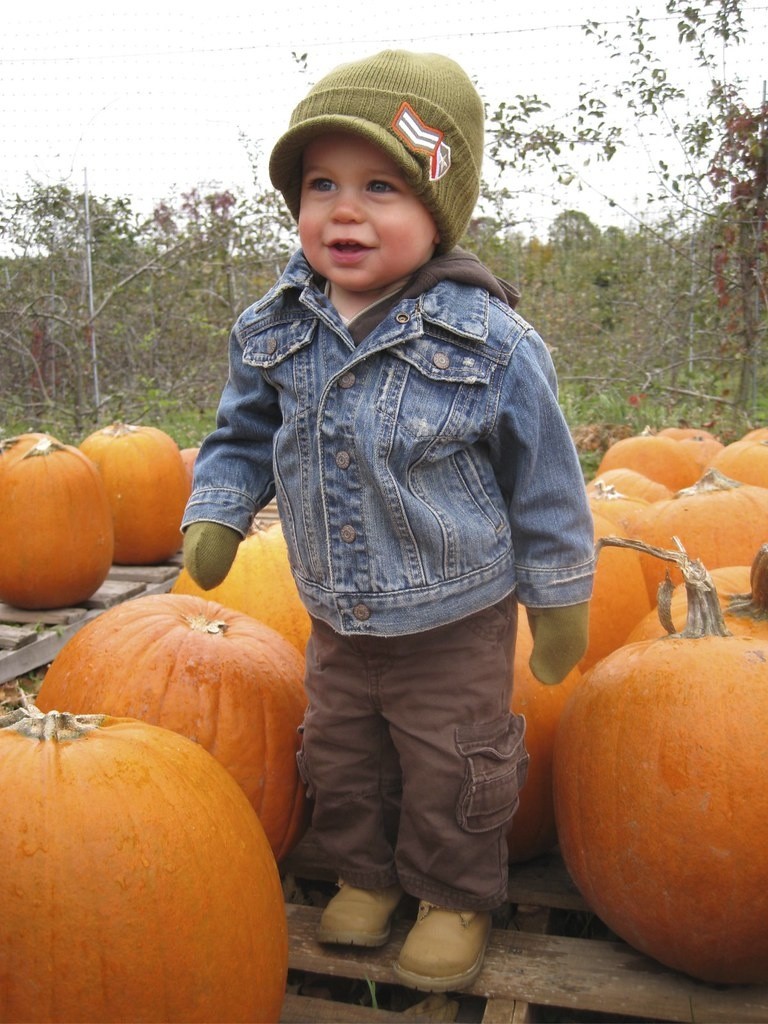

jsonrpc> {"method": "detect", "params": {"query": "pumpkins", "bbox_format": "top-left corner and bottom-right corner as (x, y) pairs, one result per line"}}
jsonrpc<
(0, 417), (768, 1024)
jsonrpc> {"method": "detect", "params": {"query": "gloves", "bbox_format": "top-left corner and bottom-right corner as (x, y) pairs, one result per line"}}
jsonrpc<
(525, 601), (590, 685)
(183, 521), (242, 591)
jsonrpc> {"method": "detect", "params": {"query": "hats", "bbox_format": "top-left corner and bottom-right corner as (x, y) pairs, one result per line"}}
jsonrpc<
(269, 49), (484, 254)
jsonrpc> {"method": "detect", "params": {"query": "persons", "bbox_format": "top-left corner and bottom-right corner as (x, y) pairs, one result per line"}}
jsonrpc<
(179, 50), (597, 991)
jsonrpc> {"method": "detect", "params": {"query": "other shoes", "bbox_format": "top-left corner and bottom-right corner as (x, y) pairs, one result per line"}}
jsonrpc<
(315, 878), (401, 946)
(393, 898), (493, 993)
